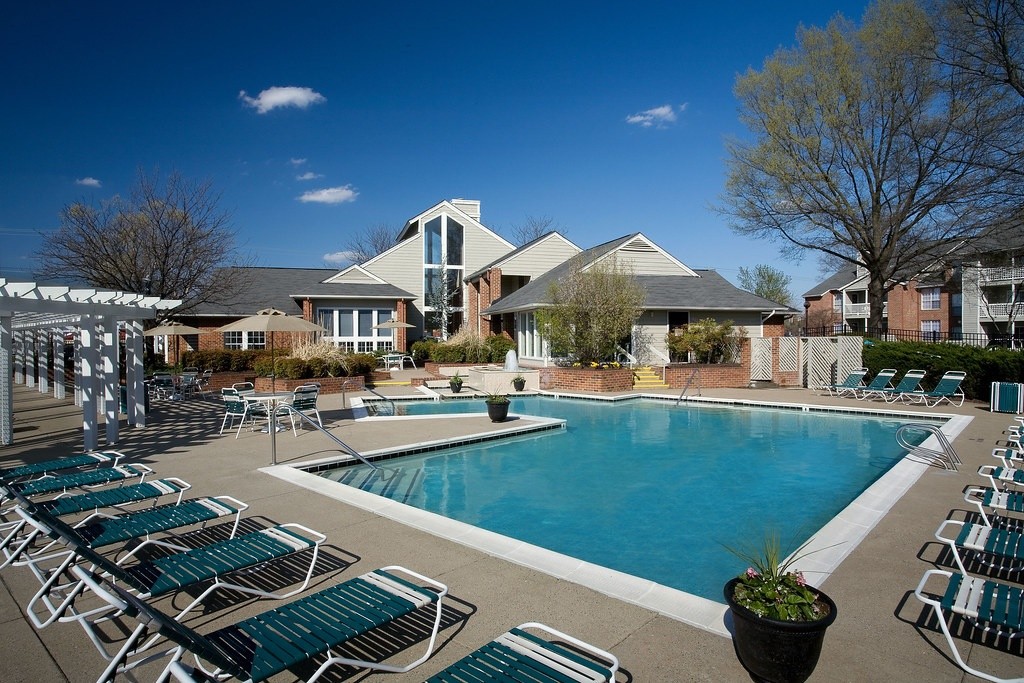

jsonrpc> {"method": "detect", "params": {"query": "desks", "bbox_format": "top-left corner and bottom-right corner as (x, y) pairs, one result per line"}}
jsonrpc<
(157, 374), (195, 399)
(381, 354), (407, 371)
(245, 392), (298, 433)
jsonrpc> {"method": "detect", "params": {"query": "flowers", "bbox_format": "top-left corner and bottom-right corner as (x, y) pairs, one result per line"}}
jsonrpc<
(705, 525), (846, 622)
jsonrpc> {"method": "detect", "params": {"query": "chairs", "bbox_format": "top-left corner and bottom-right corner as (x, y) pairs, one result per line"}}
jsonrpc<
(219, 387), (259, 438)
(232, 382), (271, 426)
(0, 449), (126, 507)
(861, 369), (927, 403)
(900, 371), (967, 408)
(13, 504), (327, 663)
(913, 415), (1024, 683)
(370, 349), (417, 370)
(811, 367), (869, 397)
(0, 462), (151, 515)
(836, 369), (898, 400)
(146, 367), (215, 402)
(0, 477), (193, 559)
(0, 479), (251, 598)
(277, 381), (328, 429)
(274, 384), (324, 437)
(72, 563), (449, 683)
(419, 622), (620, 683)
(989, 380), (1024, 414)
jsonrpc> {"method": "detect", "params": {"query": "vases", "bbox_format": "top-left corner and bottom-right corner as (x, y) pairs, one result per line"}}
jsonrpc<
(724, 576), (838, 683)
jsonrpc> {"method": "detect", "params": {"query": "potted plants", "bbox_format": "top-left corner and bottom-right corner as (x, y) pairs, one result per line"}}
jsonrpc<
(481, 386), (511, 423)
(510, 373), (528, 391)
(449, 369), (463, 393)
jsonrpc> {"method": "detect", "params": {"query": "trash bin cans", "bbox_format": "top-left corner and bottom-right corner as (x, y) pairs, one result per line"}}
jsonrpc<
(144, 385), (152, 417)
(119, 385), (127, 414)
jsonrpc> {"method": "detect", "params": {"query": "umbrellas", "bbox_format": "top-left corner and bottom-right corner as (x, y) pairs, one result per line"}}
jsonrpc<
(214, 307), (327, 410)
(370, 318), (416, 363)
(142, 321), (209, 372)
